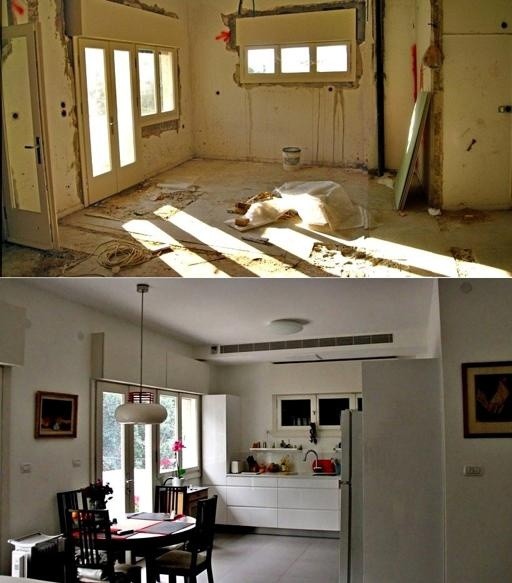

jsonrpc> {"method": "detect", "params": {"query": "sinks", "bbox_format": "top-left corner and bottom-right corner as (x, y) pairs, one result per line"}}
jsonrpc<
(284, 471), (312, 476)
(312, 474), (337, 476)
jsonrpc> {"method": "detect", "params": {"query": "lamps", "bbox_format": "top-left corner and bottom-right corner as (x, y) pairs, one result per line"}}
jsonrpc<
(267, 319), (303, 336)
(115, 284), (168, 425)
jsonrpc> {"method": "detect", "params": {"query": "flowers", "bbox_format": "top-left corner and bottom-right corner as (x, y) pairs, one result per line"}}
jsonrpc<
(172, 440), (186, 479)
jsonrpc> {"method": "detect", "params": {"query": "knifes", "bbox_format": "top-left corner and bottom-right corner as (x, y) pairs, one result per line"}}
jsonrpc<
(246, 455), (254, 464)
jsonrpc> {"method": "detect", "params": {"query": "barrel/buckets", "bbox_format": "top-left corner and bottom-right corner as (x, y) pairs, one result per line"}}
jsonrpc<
(281, 146), (302, 172)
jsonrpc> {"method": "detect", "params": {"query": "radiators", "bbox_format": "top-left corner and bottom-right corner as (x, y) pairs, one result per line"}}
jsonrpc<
(7, 532), (67, 583)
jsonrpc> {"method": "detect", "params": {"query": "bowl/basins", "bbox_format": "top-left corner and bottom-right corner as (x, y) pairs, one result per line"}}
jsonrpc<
(292, 416), (307, 425)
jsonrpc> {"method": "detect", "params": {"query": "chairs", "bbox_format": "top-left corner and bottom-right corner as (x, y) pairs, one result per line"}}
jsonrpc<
(56, 485), (218, 583)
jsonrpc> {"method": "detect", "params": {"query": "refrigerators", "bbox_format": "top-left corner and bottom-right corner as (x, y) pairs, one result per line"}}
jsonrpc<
(338, 407), (363, 582)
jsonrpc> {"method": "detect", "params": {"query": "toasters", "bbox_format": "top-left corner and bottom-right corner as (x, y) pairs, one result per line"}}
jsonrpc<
(231, 459), (245, 472)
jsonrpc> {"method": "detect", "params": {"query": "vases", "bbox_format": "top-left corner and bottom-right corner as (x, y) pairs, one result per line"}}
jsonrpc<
(172, 477), (184, 490)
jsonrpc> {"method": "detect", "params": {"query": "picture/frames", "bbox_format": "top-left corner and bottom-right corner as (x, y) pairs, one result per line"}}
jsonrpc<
(461, 360), (512, 439)
(34, 391), (78, 438)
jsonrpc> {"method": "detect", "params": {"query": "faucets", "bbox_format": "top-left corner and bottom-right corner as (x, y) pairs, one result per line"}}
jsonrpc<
(330, 458), (340, 473)
(303, 449), (323, 470)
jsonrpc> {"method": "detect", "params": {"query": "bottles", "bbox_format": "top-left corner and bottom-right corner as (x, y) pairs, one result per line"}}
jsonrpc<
(263, 438), (297, 447)
(281, 455), (288, 471)
(259, 463), (265, 471)
